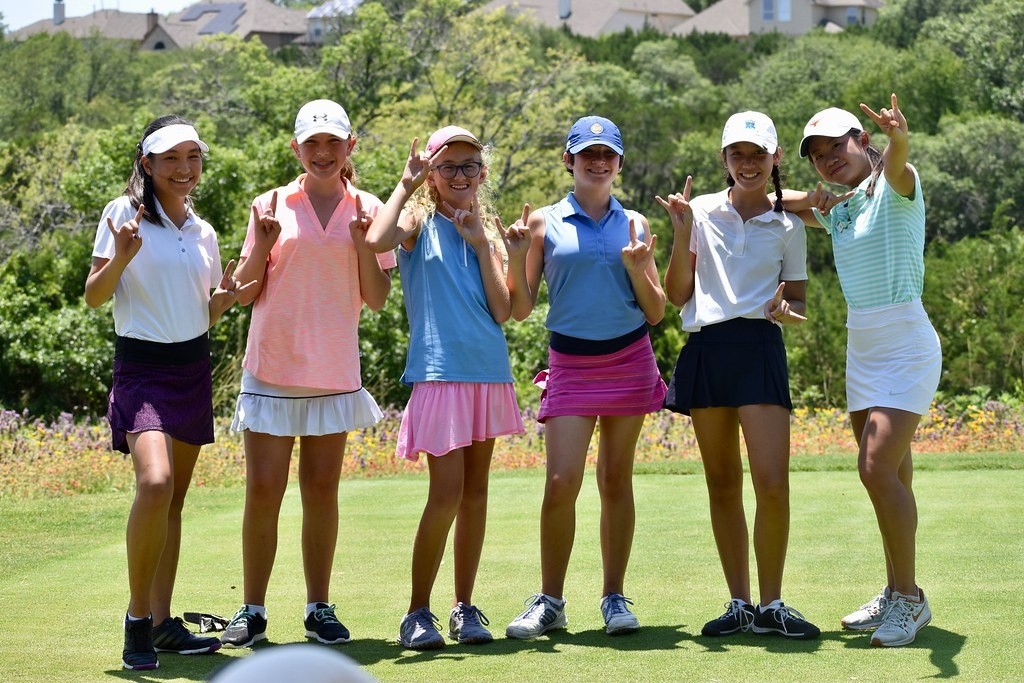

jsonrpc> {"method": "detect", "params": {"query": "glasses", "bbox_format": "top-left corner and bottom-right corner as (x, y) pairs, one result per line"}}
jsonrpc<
(430, 162), (483, 180)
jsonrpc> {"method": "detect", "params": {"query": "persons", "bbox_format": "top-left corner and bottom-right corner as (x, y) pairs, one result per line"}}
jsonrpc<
(768, 92), (942, 648)
(495, 115), (670, 639)
(364, 126), (524, 651)
(84, 114), (258, 670)
(655, 111), (821, 640)
(220, 98), (396, 650)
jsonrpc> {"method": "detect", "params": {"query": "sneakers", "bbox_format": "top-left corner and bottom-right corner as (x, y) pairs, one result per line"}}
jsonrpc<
(220, 605), (267, 649)
(701, 600), (756, 636)
(840, 586), (893, 630)
(152, 617), (221, 655)
(396, 607), (446, 648)
(600, 592), (640, 635)
(504, 592), (569, 640)
(447, 602), (493, 645)
(751, 601), (821, 639)
(869, 587), (932, 646)
(121, 610), (159, 670)
(304, 603), (351, 644)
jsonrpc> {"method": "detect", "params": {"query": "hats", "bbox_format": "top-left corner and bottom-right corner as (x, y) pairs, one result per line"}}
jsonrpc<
(721, 111), (778, 154)
(425, 126), (483, 157)
(142, 124), (209, 156)
(798, 108), (863, 158)
(294, 99), (350, 145)
(566, 116), (624, 155)
